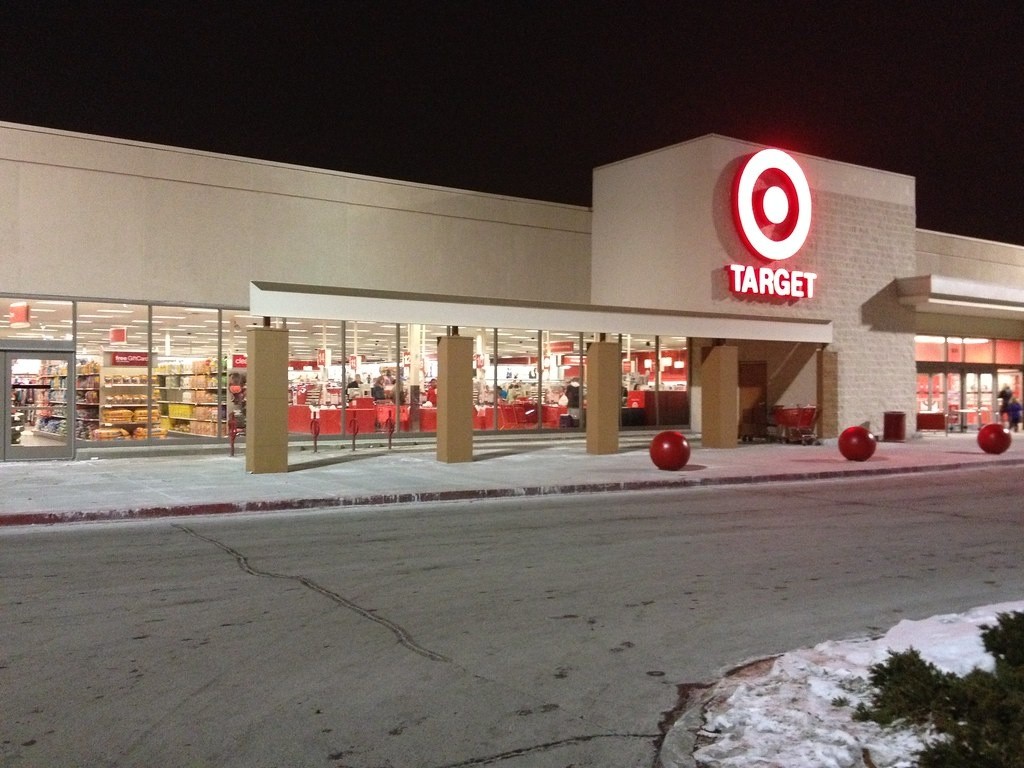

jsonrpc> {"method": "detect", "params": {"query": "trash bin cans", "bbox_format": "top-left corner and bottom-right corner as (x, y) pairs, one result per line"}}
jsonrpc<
(883, 411), (906, 443)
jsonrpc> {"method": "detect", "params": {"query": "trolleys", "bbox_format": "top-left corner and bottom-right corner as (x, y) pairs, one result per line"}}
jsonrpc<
(741, 403), (822, 446)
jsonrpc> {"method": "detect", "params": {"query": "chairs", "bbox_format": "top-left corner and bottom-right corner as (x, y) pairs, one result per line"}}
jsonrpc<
(499, 403), (519, 431)
(512, 404), (532, 430)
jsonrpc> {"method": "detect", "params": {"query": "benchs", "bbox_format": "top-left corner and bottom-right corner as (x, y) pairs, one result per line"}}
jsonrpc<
(375, 405), (412, 432)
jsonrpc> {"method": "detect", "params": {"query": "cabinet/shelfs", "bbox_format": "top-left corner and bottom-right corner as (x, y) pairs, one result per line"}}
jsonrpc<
(31, 350), (159, 443)
(151, 371), (229, 439)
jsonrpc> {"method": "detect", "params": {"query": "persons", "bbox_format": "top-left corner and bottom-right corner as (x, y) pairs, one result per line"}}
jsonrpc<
(10, 378), (37, 408)
(495, 384), (527, 402)
(996, 383), (1023, 432)
(565, 377), (580, 420)
(344, 370), (437, 409)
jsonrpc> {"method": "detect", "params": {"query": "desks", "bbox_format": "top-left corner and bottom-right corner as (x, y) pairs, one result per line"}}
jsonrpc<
(420, 401), (437, 432)
(945, 408), (991, 436)
(289, 405), (376, 434)
